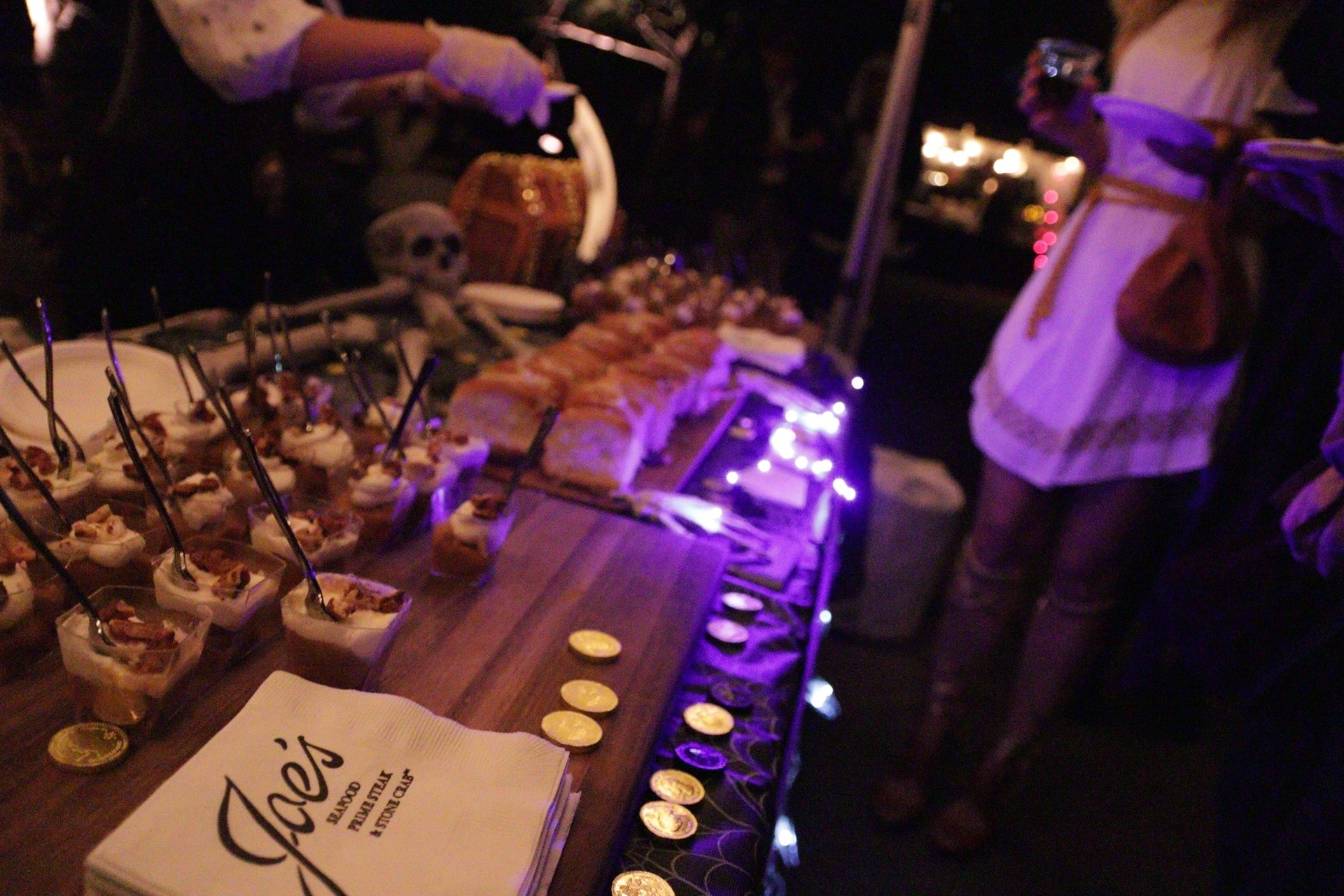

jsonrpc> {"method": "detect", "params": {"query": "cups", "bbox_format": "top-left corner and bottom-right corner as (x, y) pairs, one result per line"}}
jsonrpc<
(1037, 38), (1099, 104)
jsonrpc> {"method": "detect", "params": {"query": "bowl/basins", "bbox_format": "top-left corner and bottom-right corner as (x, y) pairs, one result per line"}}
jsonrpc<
(0, 373), (516, 734)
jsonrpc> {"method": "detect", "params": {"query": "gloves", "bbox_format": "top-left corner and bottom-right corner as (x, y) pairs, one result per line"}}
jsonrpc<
(423, 21), (554, 129)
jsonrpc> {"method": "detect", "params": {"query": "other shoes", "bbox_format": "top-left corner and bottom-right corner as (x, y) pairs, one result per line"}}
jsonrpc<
(874, 736), (1019, 859)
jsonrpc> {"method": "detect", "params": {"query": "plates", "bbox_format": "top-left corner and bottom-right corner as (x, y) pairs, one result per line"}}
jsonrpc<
(456, 284), (564, 324)
(1241, 139), (1343, 172)
(1089, 92), (1221, 150)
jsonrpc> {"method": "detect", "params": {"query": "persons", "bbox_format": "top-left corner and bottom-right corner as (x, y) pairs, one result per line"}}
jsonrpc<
(874, 0), (1344, 844)
(32, 0), (554, 334)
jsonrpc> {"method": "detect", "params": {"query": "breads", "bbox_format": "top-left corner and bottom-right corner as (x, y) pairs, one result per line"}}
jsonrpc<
(443, 307), (732, 495)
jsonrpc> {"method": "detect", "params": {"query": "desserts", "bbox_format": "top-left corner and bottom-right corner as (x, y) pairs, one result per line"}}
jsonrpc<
(430, 487), (512, 582)
(0, 370), (489, 737)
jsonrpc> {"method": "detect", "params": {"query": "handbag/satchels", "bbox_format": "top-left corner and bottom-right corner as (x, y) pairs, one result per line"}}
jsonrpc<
(1119, 206), (1265, 369)
